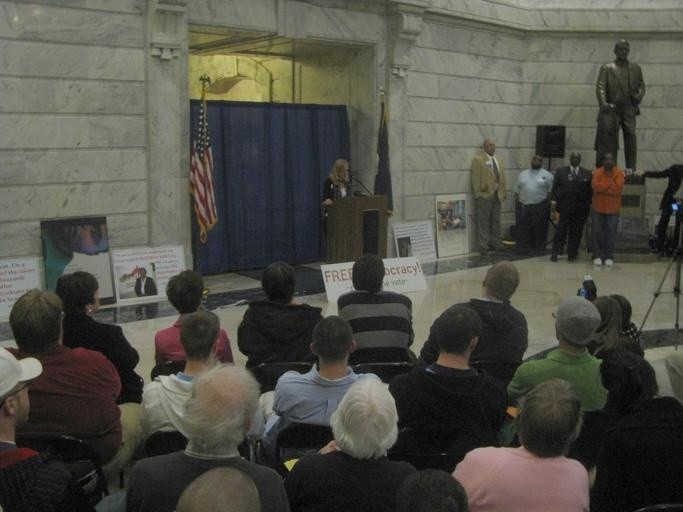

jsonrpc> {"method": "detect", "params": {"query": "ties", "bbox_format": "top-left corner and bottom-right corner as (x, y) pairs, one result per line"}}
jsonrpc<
(491, 158), (500, 180)
(573, 168), (577, 181)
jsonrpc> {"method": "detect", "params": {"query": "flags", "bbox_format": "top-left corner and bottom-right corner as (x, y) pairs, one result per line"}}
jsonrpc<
(189, 92), (218, 244)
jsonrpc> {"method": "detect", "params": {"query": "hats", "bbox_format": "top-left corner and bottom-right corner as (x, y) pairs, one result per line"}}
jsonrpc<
(556, 297), (601, 346)
(0, 348), (42, 398)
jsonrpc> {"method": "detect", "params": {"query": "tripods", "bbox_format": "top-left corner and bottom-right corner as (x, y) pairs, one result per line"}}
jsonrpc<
(636, 217), (683, 351)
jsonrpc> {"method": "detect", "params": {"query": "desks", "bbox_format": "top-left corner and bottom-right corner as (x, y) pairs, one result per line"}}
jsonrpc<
(325, 195), (389, 263)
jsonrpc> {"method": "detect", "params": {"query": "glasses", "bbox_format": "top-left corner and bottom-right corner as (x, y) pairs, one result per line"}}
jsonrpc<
(1, 379), (31, 407)
(552, 311), (558, 318)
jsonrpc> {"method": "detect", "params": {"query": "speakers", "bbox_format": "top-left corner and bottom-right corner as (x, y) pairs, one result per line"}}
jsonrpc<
(535, 123), (565, 160)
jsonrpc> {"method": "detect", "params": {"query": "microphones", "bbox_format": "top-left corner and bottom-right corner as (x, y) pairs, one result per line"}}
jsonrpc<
(347, 174), (373, 196)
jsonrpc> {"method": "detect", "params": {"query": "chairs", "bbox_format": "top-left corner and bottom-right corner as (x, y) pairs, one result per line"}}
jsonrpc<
(150, 360), (186, 380)
(144, 424), (263, 470)
(411, 424), (507, 473)
(471, 359), (525, 377)
(572, 406), (622, 444)
(246, 362), (313, 393)
(353, 362), (413, 381)
(18, 427), (125, 496)
(274, 421), (407, 474)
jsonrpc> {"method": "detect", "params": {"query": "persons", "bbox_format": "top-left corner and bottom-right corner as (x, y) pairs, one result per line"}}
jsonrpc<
(594, 39), (645, 172)
(321, 159), (352, 240)
(506, 294), (609, 415)
(417, 260), (528, 388)
(567, 349), (683, 512)
(592, 296), (644, 360)
(388, 305), (506, 475)
(637, 165), (683, 260)
(283, 373), (416, 512)
(245, 315), (365, 469)
(54, 271), (144, 405)
(588, 154), (624, 267)
(139, 311), (225, 440)
(513, 155), (553, 256)
(451, 376), (590, 512)
(126, 361), (291, 512)
(338, 255), (417, 384)
(550, 151), (593, 263)
(237, 261), (324, 368)
(0, 346), (96, 511)
(154, 269), (227, 365)
(0, 288), (122, 466)
(134, 268), (162, 295)
(397, 468), (469, 512)
(471, 138), (508, 252)
(173, 467), (262, 512)
